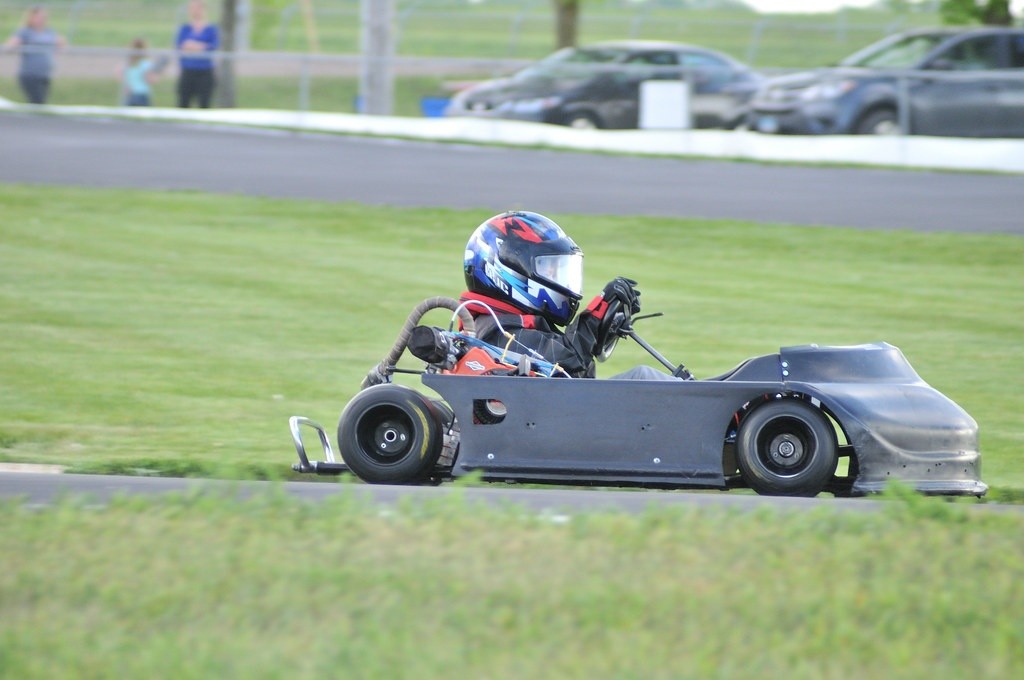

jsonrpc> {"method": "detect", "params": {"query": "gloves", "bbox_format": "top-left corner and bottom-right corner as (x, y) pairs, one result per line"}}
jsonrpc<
(600, 277), (640, 314)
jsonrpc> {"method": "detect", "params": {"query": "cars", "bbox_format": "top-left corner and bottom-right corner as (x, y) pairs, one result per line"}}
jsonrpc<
(440, 38), (767, 133)
(744, 23), (1024, 141)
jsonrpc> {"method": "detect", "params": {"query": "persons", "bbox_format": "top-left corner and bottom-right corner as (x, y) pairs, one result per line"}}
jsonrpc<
(458, 211), (684, 379)
(121, 0), (221, 109)
(0, 7), (67, 103)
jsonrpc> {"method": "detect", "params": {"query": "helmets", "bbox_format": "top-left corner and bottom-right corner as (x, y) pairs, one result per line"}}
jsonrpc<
(464, 210), (584, 327)
